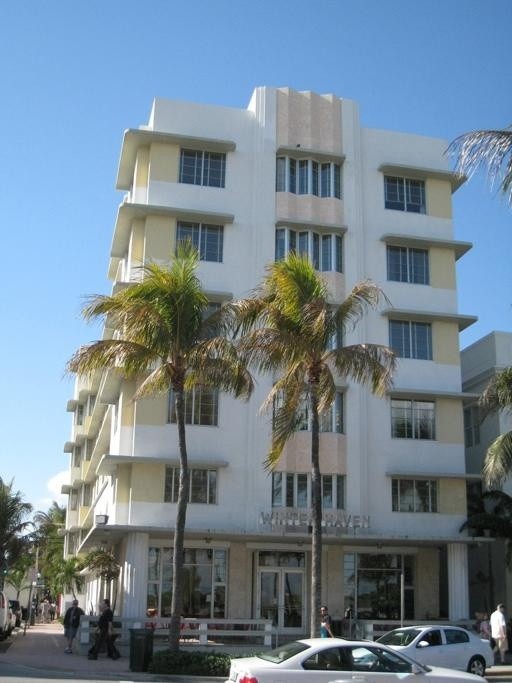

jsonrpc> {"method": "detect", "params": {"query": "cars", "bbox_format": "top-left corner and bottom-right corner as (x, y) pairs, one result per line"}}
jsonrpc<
(224, 624), (495, 683)
(0, 590), (22, 641)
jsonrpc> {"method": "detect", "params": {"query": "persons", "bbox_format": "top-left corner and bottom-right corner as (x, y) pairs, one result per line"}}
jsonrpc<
(488, 603), (509, 665)
(476, 612), (491, 640)
(39, 598), (57, 624)
(344, 603), (353, 619)
(88, 598), (121, 660)
(321, 606), (332, 638)
(63, 599), (84, 654)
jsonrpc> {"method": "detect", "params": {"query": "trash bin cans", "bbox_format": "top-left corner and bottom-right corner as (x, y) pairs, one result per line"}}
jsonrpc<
(128, 628), (153, 672)
